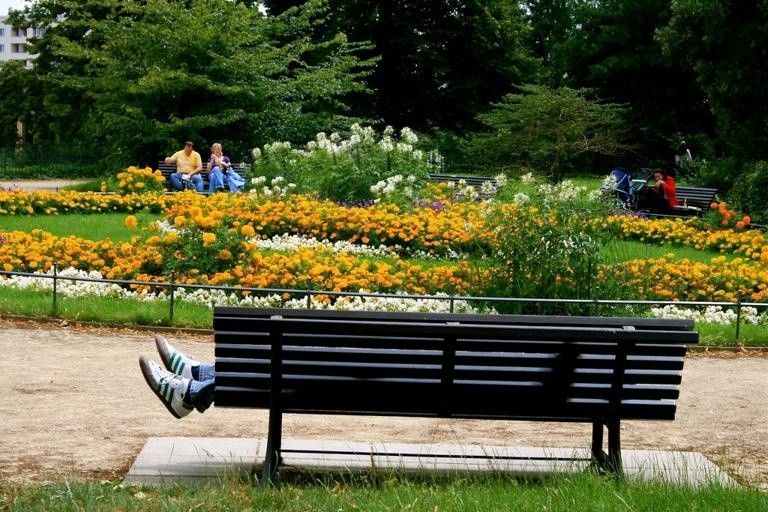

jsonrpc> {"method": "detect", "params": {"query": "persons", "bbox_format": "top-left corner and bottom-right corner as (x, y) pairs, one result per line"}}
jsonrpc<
(164, 141), (204, 192)
(678, 141), (692, 160)
(139, 333), (215, 419)
(206, 142), (238, 195)
(639, 171), (680, 212)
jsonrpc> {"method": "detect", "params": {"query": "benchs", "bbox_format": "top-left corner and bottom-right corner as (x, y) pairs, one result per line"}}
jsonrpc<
(156, 161), (251, 193)
(633, 184), (719, 219)
(427, 174), (496, 199)
(212, 307), (698, 481)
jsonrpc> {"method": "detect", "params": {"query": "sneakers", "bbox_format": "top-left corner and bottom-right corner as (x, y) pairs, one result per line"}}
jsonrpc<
(139, 355), (194, 419)
(155, 335), (200, 380)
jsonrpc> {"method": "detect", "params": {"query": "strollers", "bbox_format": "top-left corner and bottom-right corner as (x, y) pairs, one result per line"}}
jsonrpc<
(600, 170), (652, 213)
